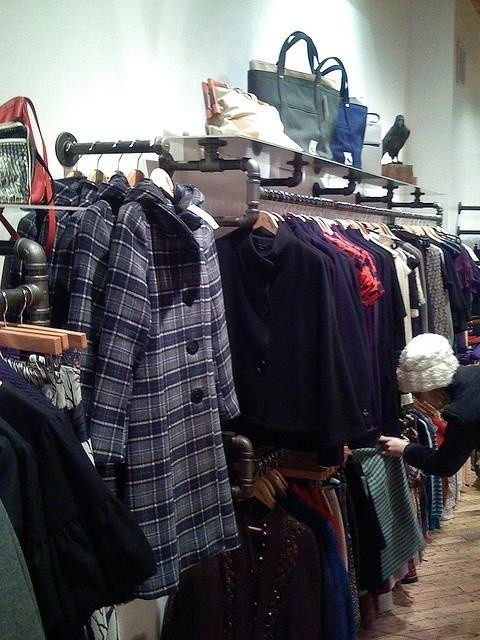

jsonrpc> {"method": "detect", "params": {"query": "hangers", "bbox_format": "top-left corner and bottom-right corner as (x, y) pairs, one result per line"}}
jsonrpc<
(64, 140), (177, 198)
(245, 455), (288, 510)
(251, 189), (465, 260)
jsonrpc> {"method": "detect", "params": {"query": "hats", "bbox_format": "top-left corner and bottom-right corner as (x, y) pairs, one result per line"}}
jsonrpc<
(398, 333), (458, 393)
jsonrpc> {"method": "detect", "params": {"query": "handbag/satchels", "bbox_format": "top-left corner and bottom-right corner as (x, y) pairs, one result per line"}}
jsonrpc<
(0, 96), (46, 204)
(202, 31), (383, 176)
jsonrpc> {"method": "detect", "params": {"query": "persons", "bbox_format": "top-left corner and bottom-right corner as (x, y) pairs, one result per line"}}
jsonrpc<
(375, 330), (480, 481)
